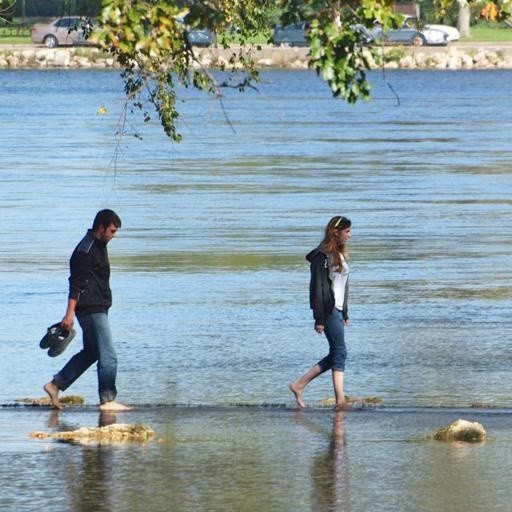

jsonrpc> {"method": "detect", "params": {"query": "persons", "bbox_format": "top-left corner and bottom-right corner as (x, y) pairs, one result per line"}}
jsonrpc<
(44, 209), (135, 412)
(46, 413), (114, 510)
(291, 408), (347, 512)
(287, 216), (351, 408)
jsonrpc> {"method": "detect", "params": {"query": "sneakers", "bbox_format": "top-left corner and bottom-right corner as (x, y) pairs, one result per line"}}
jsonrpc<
(39, 323), (77, 358)
